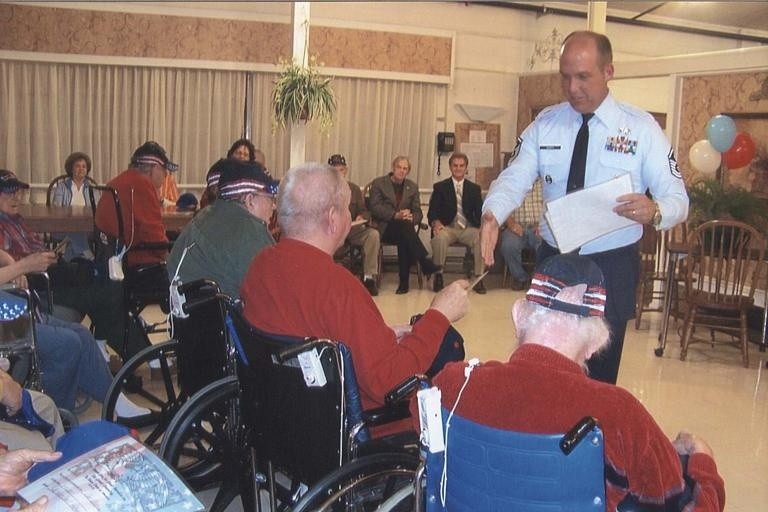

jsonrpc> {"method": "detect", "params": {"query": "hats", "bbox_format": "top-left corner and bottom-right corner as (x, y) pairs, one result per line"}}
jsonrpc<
(523, 250), (611, 320)
(129, 139), (180, 173)
(219, 158), (282, 201)
(204, 157), (241, 190)
(1, 291), (32, 327)
(0, 167), (31, 196)
(328, 153), (347, 168)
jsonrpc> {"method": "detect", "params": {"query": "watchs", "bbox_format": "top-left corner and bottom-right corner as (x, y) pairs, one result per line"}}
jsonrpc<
(650, 203), (661, 228)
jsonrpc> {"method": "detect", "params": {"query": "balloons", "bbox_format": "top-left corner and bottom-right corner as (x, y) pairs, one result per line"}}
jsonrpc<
(689, 140), (721, 173)
(721, 132), (756, 169)
(706, 115), (737, 153)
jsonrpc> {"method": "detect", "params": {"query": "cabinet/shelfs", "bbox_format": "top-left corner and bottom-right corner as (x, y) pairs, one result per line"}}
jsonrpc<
(455, 123), (500, 190)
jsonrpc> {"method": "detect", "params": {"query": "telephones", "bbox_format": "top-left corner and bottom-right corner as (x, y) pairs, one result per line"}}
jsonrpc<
(438, 132), (455, 152)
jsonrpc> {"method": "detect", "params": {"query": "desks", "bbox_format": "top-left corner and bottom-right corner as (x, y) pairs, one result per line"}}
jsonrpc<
(655, 243), (768, 356)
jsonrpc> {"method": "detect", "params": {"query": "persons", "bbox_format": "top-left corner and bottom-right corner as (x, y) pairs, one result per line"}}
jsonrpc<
(1, 140), (279, 512)
(500, 178), (544, 291)
(240, 162), (470, 438)
(329, 155), (380, 296)
(427, 152), (488, 293)
(411, 253), (724, 512)
(369, 155), (443, 295)
(479, 30), (690, 385)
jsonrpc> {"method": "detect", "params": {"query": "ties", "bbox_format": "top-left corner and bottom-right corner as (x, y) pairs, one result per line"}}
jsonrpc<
(454, 183), (470, 229)
(562, 111), (598, 199)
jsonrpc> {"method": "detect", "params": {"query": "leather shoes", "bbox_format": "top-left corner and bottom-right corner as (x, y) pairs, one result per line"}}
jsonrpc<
(420, 260), (443, 276)
(432, 272), (445, 293)
(472, 273), (487, 296)
(394, 279), (410, 296)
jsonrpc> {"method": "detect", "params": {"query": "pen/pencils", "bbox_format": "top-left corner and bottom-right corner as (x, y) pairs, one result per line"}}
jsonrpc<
(466, 269), (490, 293)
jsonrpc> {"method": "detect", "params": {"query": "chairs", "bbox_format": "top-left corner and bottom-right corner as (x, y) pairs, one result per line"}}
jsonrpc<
(45, 174), (103, 254)
(334, 239), (379, 277)
(361, 182), (428, 289)
(680, 220), (764, 368)
(431, 228), (474, 276)
(505, 225), (536, 288)
(636, 224), (696, 329)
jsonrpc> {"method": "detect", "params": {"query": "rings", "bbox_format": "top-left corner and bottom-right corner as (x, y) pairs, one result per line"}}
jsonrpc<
(632, 209), (636, 218)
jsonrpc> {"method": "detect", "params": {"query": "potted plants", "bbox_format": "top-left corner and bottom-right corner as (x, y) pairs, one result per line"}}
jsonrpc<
(270, 52), (337, 143)
(684, 172), (767, 251)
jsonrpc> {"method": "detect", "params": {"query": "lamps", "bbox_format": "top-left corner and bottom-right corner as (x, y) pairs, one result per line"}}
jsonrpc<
(459, 102), (507, 124)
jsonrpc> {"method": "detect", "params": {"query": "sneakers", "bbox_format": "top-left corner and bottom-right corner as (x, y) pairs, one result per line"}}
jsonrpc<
(117, 404), (163, 428)
(361, 276), (380, 297)
(146, 358), (184, 383)
(510, 279), (529, 291)
